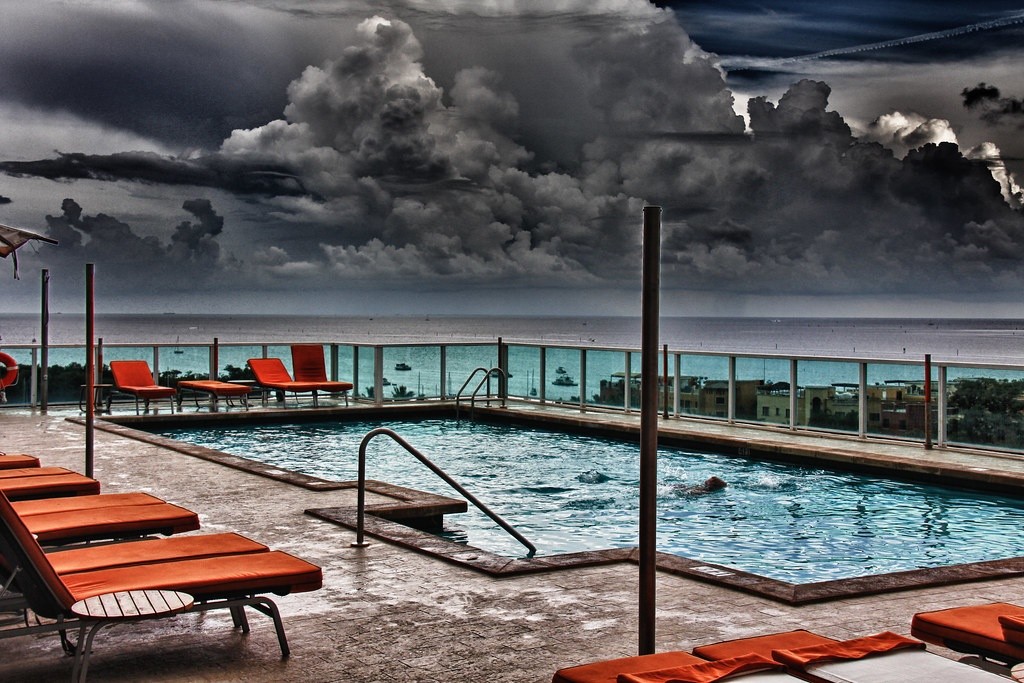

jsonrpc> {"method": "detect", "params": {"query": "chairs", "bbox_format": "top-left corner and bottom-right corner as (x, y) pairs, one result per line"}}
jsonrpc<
(0, 453), (323, 656)
(291, 344), (353, 408)
(107, 360), (177, 415)
(247, 358), (328, 410)
(551, 601), (1024, 683)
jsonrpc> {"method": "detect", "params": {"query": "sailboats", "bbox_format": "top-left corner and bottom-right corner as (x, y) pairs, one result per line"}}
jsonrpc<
(173, 335), (184, 354)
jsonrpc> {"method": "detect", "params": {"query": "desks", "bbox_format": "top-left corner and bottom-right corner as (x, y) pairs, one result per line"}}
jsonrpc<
(1011, 662), (1024, 683)
(226, 380), (257, 408)
(71, 590), (194, 683)
(79, 384), (114, 413)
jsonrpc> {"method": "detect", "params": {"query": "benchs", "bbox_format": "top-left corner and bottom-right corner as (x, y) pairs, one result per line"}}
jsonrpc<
(177, 380), (252, 412)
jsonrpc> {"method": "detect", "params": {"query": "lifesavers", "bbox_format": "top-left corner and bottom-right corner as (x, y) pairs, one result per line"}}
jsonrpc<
(0, 351), (19, 391)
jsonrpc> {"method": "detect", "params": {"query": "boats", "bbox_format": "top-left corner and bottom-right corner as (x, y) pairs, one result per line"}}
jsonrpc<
(556, 367), (565, 373)
(551, 374), (578, 386)
(491, 371), (513, 378)
(395, 363), (411, 370)
